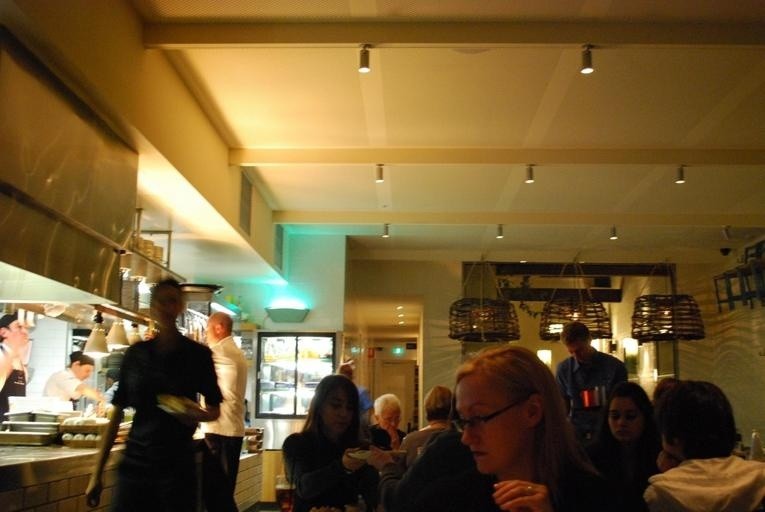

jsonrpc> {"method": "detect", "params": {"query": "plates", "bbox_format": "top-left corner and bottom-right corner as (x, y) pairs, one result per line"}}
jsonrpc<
(348, 450), (405, 460)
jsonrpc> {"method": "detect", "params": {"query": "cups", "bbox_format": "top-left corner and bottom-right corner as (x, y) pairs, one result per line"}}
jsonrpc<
(275, 475), (295, 511)
(596, 386), (605, 405)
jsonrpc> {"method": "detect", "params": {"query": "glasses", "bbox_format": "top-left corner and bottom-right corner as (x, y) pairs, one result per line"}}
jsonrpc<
(451, 395), (528, 432)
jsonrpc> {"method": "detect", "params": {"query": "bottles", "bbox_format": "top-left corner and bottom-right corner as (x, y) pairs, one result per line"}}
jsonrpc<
(583, 389), (598, 406)
(751, 428), (765, 459)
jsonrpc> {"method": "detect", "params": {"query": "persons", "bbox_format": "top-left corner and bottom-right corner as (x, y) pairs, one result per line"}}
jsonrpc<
(200, 311), (247, 512)
(103, 369), (120, 406)
(0, 312), (29, 432)
(85, 277), (224, 512)
(42, 351), (106, 417)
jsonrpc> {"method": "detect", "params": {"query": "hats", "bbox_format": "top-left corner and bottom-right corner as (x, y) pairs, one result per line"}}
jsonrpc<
(65, 351), (96, 369)
(0, 315), (17, 330)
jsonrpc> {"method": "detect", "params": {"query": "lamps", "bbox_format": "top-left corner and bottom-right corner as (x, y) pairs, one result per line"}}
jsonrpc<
(80, 306), (142, 358)
(375, 163), (385, 184)
(580, 44), (594, 73)
(381, 224), (389, 239)
(496, 223), (504, 238)
(358, 43), (372, 73)
(448, 254), (521, 343)
(630, 255), (707, 340)
(265, 305), (309, 324)
(673, 164), (685, 184)
(609, 224), (617, 240)
(525, 164), (534, 184)
(538, 254), (615, 341)
